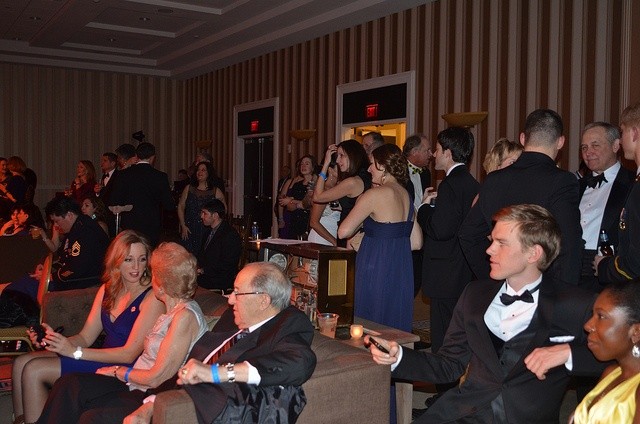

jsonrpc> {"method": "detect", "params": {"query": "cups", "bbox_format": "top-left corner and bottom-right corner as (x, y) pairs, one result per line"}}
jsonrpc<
(329, 201), (339, 210)
(317, 313), (339, 339)
(350, 324), (364, 338)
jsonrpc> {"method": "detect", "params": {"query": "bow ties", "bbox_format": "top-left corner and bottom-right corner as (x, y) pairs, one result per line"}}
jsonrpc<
(585, 173), (607, 189)
(406, 162), (426, 175)
(499, 280), (541, 305)
(103, 172), (109, 179)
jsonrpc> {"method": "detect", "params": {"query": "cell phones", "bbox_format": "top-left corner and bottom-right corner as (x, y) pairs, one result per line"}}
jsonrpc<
(27, 323), (48, 344)
(367, 336), (390, 353)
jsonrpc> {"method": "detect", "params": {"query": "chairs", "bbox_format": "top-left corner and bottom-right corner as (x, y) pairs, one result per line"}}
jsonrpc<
(1, 252), (55, 357)
(198, 220), (259, 293)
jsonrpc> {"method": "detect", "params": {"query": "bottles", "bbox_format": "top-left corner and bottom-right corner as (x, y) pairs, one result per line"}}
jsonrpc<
(240, 225), (247, 240)
(252, 222), (260, 240)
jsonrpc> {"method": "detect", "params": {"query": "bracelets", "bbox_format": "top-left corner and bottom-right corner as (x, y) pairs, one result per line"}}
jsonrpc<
(319, 172), (327, 180)
(125, 367), (134, 383)
(212, 362), (221, 385)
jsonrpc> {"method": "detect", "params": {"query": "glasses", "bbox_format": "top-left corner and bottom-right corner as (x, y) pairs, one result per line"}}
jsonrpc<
(234, 291), (263, 299)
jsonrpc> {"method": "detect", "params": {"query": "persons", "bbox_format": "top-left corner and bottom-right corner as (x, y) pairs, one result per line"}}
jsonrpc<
(0, 210), (23, 236)
(178, 161), (228, 259)
(567, 283), (640, 423)
(481, 140), (520, 172)
(483, 108), (584, 283)
(1, 205), (19, 236)
(403, 131), (434, 299)
(417, 126), (480, 408)
(0, 157), (37, 204)
(28, 223), (67, 253)
(307, 156), (343, 244)
(579, 120), (628, 259)
(47, 197), (106, 287)
(195, 197), (260, 289)
(364, 203), (596, 424)
(337, 145), (416, 334)
(620, 104), (638, 282)
(65, 160), (97, 207)
(80, 199), (110, 239)
(312, 140), (372, 247)
(95, 153), (118, 203)
(41, 243), (206, 424)
(115, 143), (138, 168)
(78, 262), (317, 424)
(364, 131), (384, 151)
(178, 169), (188, 181)
(10, 229), (165, 422)
(278, 156), (319, 238)
(104, 142), (176, 243)
(8, 205), (45, 274)
(195, 153), (213, 165)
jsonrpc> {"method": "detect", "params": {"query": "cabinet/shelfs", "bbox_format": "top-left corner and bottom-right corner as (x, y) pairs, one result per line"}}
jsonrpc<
(335, 314), (420, 424)
(245, 237), (358, 325)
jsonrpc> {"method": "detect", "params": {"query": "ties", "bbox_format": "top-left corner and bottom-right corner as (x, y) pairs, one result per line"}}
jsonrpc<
(206, 327), (249, 365)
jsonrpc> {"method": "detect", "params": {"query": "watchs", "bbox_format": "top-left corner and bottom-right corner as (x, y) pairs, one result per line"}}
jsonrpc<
(112, 366), (121, 378)
(74, 346), (83, 361)
(226, 362), (235, 382)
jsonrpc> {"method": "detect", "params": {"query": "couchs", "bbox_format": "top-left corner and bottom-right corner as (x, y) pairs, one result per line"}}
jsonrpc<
(42, 280), (390, 424)
